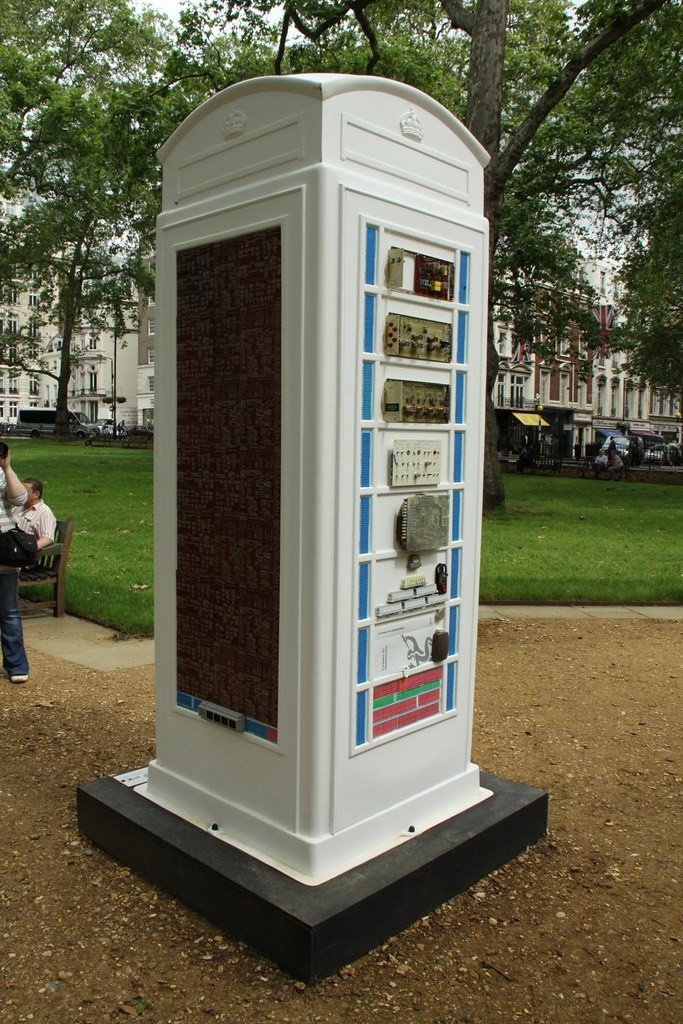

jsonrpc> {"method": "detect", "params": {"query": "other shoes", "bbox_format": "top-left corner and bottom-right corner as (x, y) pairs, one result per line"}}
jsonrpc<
(9, 674), (28, 683)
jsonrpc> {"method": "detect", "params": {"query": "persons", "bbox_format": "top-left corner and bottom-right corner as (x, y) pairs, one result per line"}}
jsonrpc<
(12, 478), (57, 573)
(626, 440), (675, 469)
(605, 435), (617, 461)
(0, 441), (30, 683)
(606, 449), (624, 481)
(516, 430), (533, 475)
(592, 448), (608, 479)
(102, 420), (129, 435)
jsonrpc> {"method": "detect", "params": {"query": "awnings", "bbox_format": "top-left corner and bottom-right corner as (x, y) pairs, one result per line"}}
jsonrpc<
(512, 412), (550, 427)
(597, 428), (623, 438)
(630, 429), (664, 438)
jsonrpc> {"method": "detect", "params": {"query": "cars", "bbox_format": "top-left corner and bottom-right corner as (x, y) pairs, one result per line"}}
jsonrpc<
(129, 426), (148, 436)
(95, 418), (117, 434)
(602, 435), (643, 461)
(643, 445), (676, 463)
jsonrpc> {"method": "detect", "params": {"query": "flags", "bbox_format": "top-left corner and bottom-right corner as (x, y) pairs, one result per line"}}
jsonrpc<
(510, 331), (533, 363)
(590, 303), (614, 359)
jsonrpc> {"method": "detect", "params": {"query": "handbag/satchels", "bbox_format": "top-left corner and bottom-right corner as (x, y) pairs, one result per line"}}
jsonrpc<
(0, 528), (38, 566)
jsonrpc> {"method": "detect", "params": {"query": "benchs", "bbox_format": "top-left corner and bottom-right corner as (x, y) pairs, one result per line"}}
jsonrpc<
(16, 515), (75, 616)
(122, 435), (149, 448)
(83, 432), (111, 448)
(581, 457), (632, 479)
(521, 455), (564, 476)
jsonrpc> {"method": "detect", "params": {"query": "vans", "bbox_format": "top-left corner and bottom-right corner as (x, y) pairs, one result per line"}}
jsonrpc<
(16, 409), (100, 439)
